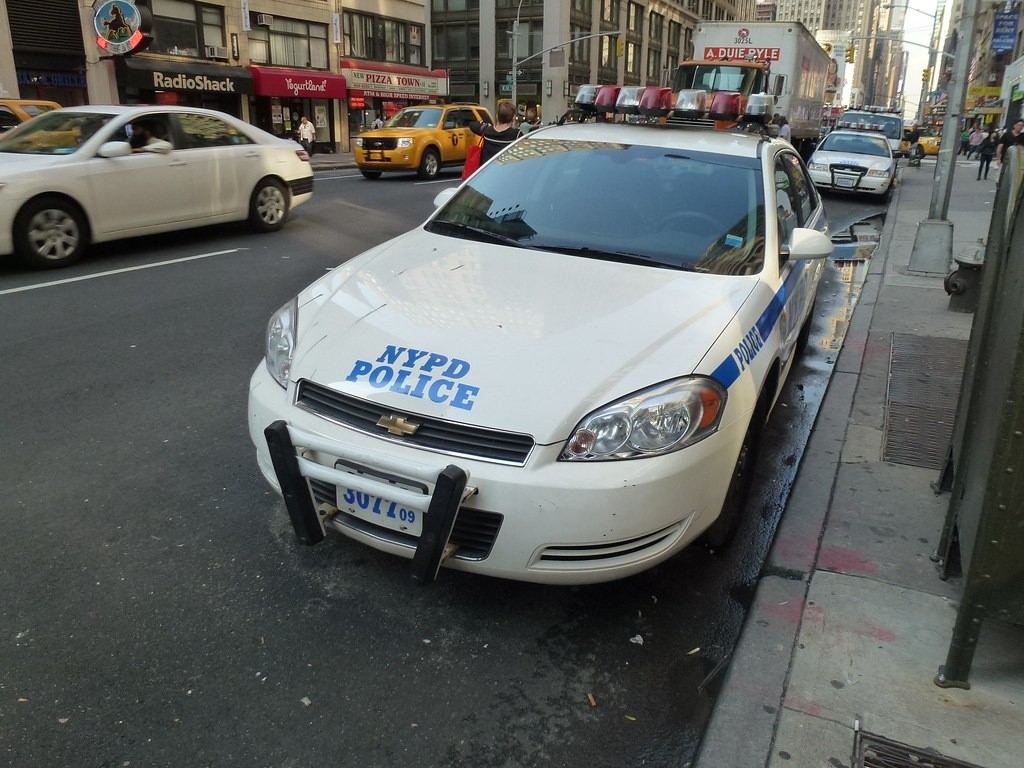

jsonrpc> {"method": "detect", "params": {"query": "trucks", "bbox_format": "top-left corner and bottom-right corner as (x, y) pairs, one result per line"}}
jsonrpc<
(661, 21), (840, 154)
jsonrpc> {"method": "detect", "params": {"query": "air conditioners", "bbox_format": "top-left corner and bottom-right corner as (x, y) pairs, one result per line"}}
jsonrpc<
(209, 46), (228, 59)
(257, 13), (273, 25)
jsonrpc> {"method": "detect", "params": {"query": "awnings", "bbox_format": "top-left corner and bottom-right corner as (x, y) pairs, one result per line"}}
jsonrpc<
(246, 66), (347, 99)
(340, 60), (449, 99)
(115, 56), (254, 95)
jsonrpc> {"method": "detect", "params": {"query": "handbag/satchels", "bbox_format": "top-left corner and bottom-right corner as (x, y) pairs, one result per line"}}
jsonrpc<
(461, 126), (488, 180)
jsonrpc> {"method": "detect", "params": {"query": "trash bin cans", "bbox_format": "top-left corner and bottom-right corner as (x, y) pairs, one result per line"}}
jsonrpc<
(909, 143), (926, 167)
(932, 147), (1023, 689)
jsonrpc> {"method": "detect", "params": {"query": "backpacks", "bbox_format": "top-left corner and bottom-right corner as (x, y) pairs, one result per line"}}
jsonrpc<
(374, 120), (381, 129)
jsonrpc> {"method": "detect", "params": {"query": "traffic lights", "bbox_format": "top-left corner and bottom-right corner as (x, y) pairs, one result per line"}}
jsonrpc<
(845, 47), (855, 63)
(616, 39), (625, 57)
(922, 69), (929, 82)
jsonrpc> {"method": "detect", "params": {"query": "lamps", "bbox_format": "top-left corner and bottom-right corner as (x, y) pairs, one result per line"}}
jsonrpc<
(546, 81), (552, 97)
(562, 81), (570, 97)
(484, 82), (489, 97)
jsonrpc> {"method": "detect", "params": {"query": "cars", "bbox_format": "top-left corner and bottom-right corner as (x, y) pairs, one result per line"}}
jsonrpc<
(250, 84), (832, 588)
(0, 98), (315, 271)
(804, 106), (941, 194)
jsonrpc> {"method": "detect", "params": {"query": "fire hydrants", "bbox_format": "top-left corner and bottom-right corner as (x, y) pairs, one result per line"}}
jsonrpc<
(943, 236), (986, 313)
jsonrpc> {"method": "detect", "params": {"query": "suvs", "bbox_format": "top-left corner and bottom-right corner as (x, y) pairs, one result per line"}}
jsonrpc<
(354, 102), (495, 180)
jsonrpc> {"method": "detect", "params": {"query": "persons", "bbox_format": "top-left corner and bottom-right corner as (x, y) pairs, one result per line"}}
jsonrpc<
(127, 120), (172, 154)
(519, 108), (543, 133)
(470, 100), (524, 165)
(911, 124), (919, 144)
(996, 119), (1024, 182)
(821, 44), (831, 54)
(292, 112), (316, 157)
(371, 113), (391, 129)
(958, 126), (1007, 181)
(80, 118), (104, 136)
(777, 116), (791, 143)
(824, 59), (840, 102)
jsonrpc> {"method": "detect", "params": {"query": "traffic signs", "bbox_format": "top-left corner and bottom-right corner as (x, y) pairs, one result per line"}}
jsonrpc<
(507, 70), (524, 76)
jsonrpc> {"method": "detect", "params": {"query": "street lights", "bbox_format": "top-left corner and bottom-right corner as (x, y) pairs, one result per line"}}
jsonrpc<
(849, 4), (988, 274)
